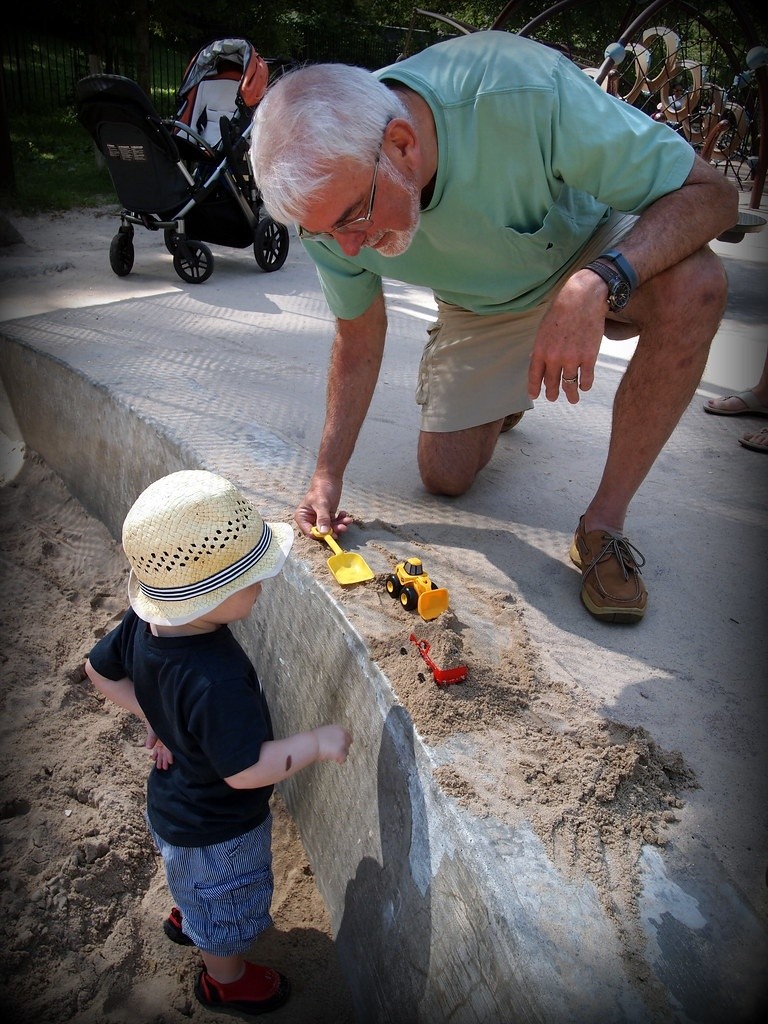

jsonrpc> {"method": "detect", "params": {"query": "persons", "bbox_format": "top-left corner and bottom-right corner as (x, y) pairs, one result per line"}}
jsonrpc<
(251, 31), (740, 619)
(85, 470), (356, 1016)
(703, 350), (768, 450)
(660, 84), (688, 136)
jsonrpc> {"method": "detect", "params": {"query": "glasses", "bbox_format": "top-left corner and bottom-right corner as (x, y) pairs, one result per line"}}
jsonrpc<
(299, 117), (393, 241)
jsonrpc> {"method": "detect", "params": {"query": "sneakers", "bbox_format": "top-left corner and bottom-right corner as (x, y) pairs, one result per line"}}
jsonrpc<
(568, 514), (648, 623)
(501, 412), (523, 432)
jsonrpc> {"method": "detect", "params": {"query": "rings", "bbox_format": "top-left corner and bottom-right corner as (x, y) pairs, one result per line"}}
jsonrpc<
(561, 375), (578, 383)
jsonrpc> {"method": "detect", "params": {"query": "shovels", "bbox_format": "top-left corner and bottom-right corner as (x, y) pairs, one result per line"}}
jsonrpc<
(312, 525), (374, 585)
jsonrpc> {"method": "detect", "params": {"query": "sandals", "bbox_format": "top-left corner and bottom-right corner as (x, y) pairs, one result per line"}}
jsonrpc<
(738, 426), (768, 451)
(703, 387), (768, 417)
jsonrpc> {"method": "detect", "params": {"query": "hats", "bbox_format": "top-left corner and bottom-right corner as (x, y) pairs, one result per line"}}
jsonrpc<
(121, 469), (294, 627)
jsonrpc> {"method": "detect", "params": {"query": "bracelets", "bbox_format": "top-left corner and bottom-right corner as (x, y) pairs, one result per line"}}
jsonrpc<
(595, 250), (638, 291)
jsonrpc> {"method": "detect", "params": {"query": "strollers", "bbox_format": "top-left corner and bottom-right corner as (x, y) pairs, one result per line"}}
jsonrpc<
(70, 37), (289, 285)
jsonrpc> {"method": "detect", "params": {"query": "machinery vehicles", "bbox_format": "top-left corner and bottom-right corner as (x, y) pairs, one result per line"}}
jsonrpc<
(410, 631), (469, 688)
(385, 557), (449, 621)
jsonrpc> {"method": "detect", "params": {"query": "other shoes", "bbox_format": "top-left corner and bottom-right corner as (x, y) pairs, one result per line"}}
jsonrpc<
(163, 908), (195, 947)
(195, 957), (291, 1011)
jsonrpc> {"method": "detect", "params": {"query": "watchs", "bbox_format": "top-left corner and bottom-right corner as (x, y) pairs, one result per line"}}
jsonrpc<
(580, 261), (631, 313)
